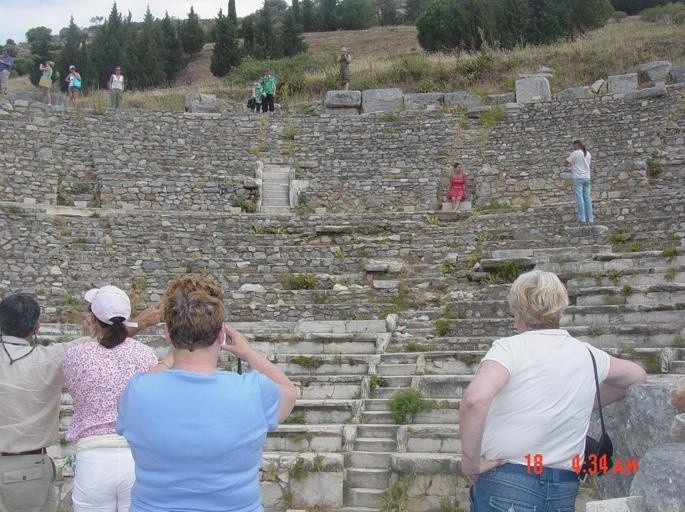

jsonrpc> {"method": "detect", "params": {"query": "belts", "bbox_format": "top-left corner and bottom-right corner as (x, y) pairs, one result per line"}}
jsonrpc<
(2, 448), (47, 456)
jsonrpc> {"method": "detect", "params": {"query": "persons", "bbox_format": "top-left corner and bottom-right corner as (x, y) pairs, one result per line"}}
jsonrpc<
(260, 72), (277, 112)
(565, 140), (595, 222)
(448, 162), (467, 212)
(115, 274), (296, 512)
(457, 270), (647, 512)
(38, 61), (55, 106)
(0, 48), (14, 96)
(250, 75), (265, 113)
(0, 294), (168, 512)
(62, 284), (159, 512)
(336, 47), (352, 90)
(108, 66), (125, 106)
(64, 64), (82, 106)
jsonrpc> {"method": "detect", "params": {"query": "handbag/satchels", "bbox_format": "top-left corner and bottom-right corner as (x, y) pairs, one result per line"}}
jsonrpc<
(73, 75), (81, 89)
(247, 98), (255, 108)
(582, 432), (613, 472)
(39, 77), (52, 89)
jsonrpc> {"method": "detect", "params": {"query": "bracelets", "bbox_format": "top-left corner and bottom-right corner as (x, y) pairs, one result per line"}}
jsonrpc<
(157, 360), (172, 369)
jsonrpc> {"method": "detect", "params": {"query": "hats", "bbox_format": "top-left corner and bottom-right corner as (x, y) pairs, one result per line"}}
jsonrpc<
(84, 286), (139, 328)
